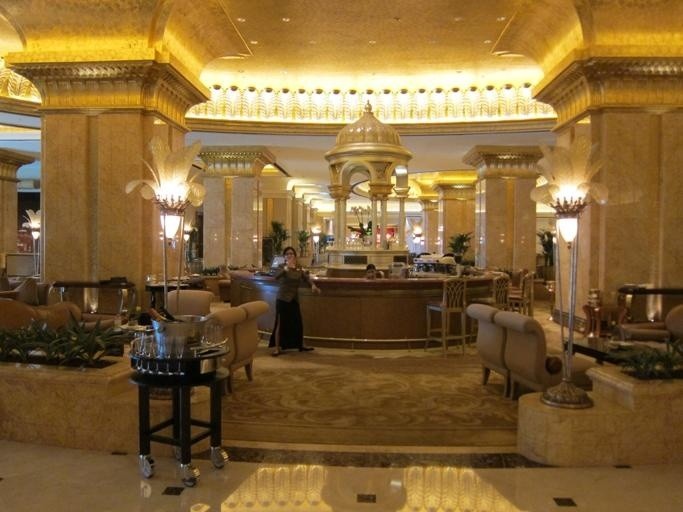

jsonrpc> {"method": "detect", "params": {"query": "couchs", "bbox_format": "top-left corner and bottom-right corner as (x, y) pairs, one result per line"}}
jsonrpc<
(1, 297), (124, 357)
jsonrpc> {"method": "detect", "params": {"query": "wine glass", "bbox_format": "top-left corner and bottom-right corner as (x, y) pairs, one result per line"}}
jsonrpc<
(132, 331), (188, 376)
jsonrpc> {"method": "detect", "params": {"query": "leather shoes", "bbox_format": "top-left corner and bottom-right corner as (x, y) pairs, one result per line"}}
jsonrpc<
(299, 347), (314, 351)
(271, 350), (280, 356)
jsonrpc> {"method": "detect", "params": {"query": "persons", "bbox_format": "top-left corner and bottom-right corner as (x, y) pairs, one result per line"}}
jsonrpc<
(365, 263), (377, 279)
(267, 246), (322, 357)
(15, 226), (34, 254)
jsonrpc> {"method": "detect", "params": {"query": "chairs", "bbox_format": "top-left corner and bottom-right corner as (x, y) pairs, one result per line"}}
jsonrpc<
(0, 268), (50, 306)
(467, 302), (602, 400)
(166, 290), (269, 396)
(424, 269), (534, 353)
(617, 304), (683, 353)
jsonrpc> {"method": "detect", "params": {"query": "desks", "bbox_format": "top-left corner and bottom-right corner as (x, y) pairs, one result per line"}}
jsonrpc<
(144, 281), (190, 310)
(128, 336), (232, 487)
(582, 304), (624, 338)
(52, 280), (137, 318)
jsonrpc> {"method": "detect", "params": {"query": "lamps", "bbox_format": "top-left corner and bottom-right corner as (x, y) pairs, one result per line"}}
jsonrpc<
(124, 135), (208, 318)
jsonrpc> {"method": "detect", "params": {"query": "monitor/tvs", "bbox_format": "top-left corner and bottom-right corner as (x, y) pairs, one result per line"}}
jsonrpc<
(270, 255), (287, 269)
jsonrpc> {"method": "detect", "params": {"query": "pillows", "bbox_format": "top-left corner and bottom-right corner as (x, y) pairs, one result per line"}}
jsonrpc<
(12, 277), (39, 305)
(624, 328), (670, 343)
(77, 318), (115, 333)
